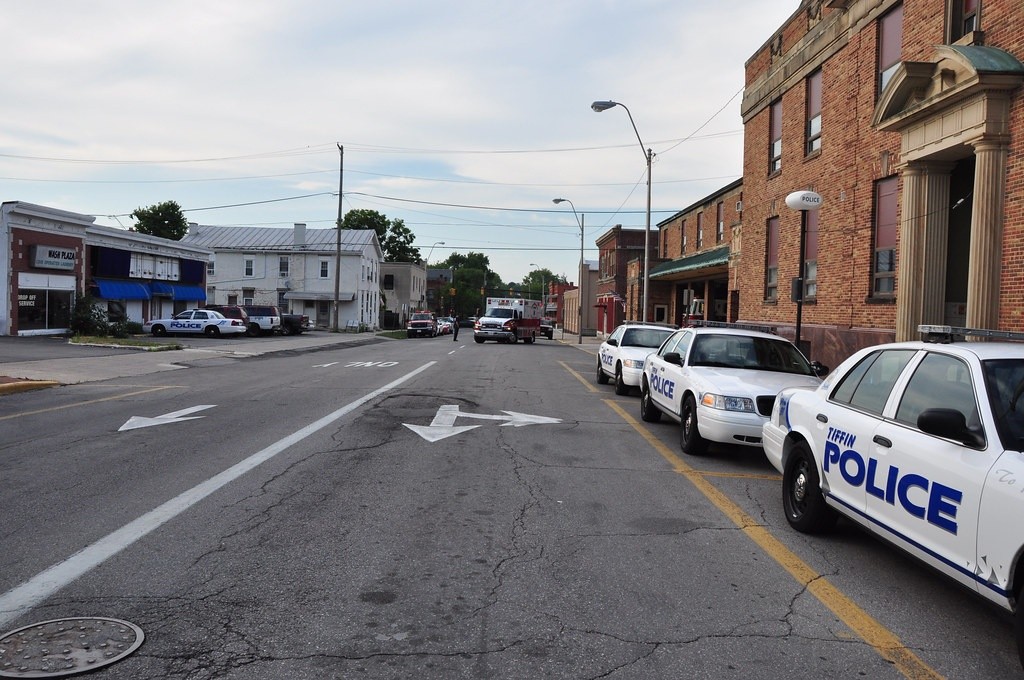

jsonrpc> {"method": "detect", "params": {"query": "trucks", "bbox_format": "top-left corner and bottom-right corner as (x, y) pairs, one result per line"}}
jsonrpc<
(473, 297), (543, 345)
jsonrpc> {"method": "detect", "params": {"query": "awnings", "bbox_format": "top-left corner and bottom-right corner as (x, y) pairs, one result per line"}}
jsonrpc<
(94, 278), (153, 301)
(149, 282), (207, 301)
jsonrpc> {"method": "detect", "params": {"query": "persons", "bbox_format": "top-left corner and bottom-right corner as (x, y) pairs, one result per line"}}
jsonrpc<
(453, 316), (461, 341)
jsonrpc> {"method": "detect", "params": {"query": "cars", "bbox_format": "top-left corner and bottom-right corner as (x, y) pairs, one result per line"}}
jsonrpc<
(468, 317), (477, 327)
(142, 308), (247, 338)
(640, 314), (835, 455)
(595, 320), (688, 395)
(540, 318), (554, 339)
(436, 316), (455, 335)
(761, 323), (1024, 663)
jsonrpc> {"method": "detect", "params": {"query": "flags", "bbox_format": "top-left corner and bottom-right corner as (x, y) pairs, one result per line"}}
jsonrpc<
(609, 289), (626, 312)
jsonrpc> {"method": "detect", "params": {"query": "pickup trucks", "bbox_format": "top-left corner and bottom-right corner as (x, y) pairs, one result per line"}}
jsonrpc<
(278, 307), (312, 335)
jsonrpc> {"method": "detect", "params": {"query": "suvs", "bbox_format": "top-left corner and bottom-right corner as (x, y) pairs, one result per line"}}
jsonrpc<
(234, 304), (286, 337)
(199, 304), (250, 332)
(406, 311), (436, 338)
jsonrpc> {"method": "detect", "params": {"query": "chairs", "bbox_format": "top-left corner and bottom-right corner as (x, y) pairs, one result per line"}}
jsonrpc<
(934, 381), (973, 417)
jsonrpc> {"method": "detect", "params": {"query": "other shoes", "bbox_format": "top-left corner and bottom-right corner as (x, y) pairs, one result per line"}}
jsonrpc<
(453, 340), (458, 341)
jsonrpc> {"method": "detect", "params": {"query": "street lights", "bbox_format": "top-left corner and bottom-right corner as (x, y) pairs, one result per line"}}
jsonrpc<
(529, 263), (545, 315)
(423, 241), (446, 312)
(553, 197), (585, 343)
(591, 99), (653, 322)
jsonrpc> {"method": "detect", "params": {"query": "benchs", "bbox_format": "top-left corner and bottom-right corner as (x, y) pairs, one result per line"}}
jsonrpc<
(346, 320), (360, 334)
